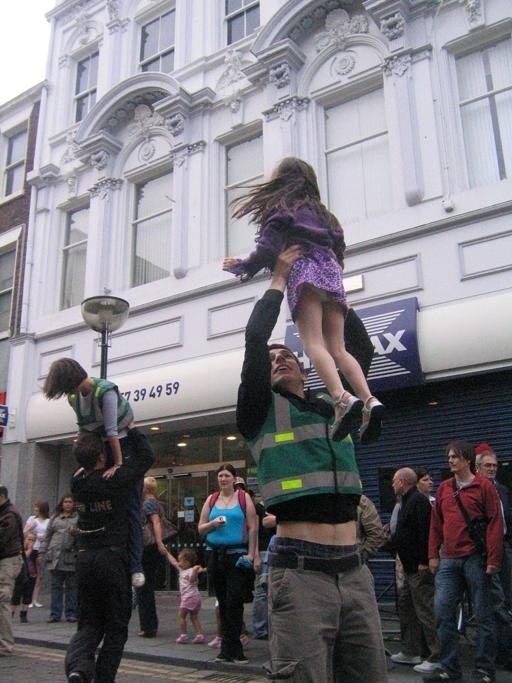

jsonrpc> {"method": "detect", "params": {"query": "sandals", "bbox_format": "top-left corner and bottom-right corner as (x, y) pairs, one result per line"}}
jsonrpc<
(330, 390), (383, 444)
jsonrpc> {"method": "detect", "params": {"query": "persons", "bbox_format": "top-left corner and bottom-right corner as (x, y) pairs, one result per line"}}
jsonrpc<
(64, 417), (154, 682)
(233, 241), (389, 682)
(0, 486), (79, 654)
(133, 463), (277, 664)
(358, 440), (512, 683)
(43, 357), (158, 588)
(219, 155), (385, 443)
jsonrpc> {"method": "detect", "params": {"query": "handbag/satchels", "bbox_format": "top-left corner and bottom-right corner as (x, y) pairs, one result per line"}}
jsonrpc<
(469, 518), (487, 556)
(141, 500), (177, 545)
(13, 573), (36, 605)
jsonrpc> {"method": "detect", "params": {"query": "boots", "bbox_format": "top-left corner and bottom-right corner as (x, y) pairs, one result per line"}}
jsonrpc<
(20, 611), (28, 623)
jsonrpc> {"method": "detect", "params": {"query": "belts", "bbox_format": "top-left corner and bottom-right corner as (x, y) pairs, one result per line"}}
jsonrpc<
(268, 552), (367, 573)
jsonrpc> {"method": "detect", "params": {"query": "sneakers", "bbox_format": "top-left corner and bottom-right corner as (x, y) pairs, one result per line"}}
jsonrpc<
(177, 635), (249, 664)
(391, 652), (495, 683)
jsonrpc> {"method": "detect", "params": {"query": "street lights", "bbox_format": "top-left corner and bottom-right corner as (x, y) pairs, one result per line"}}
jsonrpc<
(81, 285), (131, 381)
